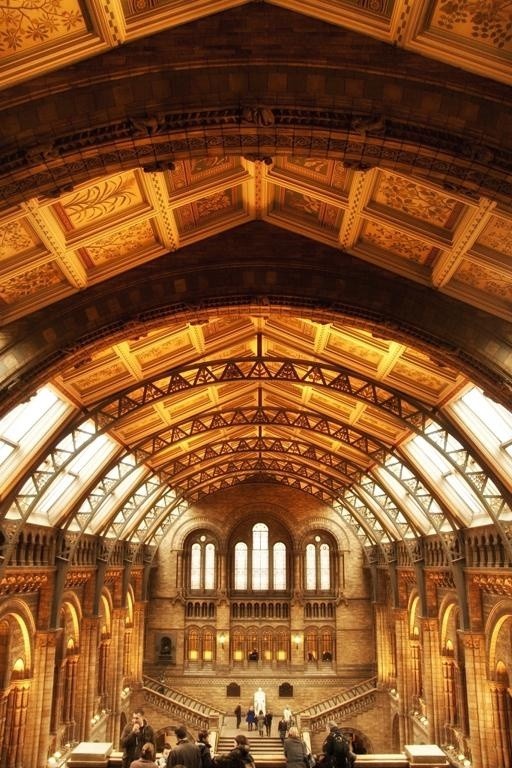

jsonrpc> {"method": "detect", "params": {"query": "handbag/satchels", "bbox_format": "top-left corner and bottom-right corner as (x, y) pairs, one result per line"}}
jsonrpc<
(305, 753), (316, 768)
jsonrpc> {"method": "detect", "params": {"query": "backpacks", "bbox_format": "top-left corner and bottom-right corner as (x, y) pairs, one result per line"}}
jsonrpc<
(331, 732), (349, 757)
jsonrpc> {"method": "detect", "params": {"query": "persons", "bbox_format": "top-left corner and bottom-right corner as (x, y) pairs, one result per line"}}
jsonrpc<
(256, 710), (266, 737)
(246, 706), (255, 731)
(286, 715), (296, 732)
(121, 707), (256, 767)
(264, 709), (273, 736)
(236, 702), (243, 729)
(283, 725), (311, 767)
(323, 720), (355, 767)
(283, 705), (292, 722)
(277, 716), (287, 739)
